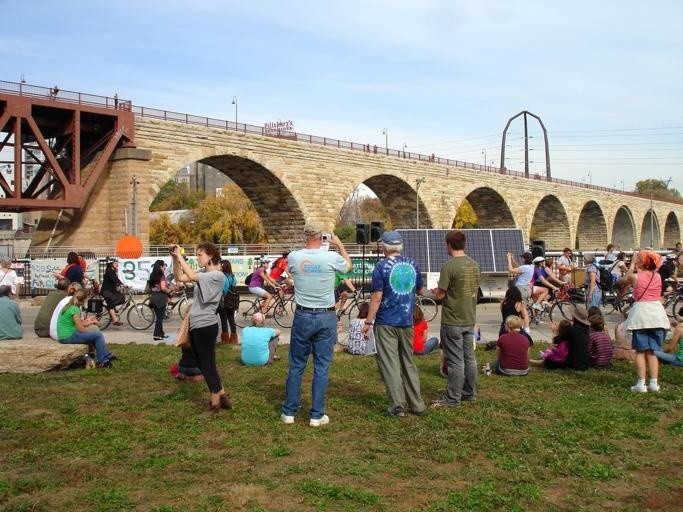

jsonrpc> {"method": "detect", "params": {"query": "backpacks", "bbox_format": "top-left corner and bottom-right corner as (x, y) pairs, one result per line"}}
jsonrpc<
(599, 267), (613, 291)
(244, 273), (253, 285)
(225, 276), (240, 312)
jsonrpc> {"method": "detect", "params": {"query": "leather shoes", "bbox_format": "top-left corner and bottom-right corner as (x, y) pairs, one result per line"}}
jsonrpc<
(154, 337), (164, 341)
(161, 334), (170, 338)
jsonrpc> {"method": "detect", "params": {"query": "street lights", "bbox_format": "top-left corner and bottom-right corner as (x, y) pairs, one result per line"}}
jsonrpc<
(19, 73), (27, 94)
(230, 97), (241, 130)
(128, 172), (138, 234)
(413, 175), (426, 229)
(381, 127), (390, 156)
(482, 148), (488, 172)
(588, 172), (592, 188)
(489, 160), (494, 173)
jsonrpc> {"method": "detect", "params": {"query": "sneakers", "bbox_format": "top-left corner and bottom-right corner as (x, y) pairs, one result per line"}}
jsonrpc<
(383, 408), (405, 417)
(532, 303), (544, 312)
(647, 384), (661, 392)
(309, 415), (329, 426)
(280, 413), (295, 424)
(630, 384), (648, 393)
(408, 406), (424, 416)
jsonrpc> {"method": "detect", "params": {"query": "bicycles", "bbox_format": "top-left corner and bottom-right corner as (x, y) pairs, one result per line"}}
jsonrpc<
(228, 287), (298, 328)
(410, 295), (440, 323)
(79, 287), (155, 330)
(519, 255), (681, 323)
(327, 291), (369, 323)
(141, 282), (197, 322)
(253, 291), (301, 315)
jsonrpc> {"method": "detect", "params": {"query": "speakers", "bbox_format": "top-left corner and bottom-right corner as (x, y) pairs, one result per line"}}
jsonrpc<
(356, 224), (369, 244)
(371, 222), (384, 242)
(532, 240), (545, 260)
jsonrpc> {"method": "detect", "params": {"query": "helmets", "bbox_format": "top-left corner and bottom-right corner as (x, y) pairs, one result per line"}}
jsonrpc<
(667, 253), (677, 261)
(533, 256), (546, 263)
(259, 258), (272, 263)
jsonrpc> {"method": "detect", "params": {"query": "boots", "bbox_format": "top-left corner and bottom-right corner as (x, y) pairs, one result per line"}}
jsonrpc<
(228, 333), (238, 343)
(218, 331), (230, 345)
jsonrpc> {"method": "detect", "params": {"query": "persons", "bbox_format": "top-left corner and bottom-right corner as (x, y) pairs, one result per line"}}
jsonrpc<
(149, 222), (682, 426)
(1, 252), (127, 369)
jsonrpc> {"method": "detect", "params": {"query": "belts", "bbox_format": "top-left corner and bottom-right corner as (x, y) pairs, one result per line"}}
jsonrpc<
(297, 305), (336, 312)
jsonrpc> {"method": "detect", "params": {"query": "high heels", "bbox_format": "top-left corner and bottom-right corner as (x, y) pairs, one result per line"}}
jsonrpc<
(200, 401), (221, 420)
(220, 393), (233, 410)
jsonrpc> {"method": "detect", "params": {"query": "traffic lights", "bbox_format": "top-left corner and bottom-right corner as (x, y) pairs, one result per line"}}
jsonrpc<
(369, 223), (383, 242)
(354, 225), (367, 245)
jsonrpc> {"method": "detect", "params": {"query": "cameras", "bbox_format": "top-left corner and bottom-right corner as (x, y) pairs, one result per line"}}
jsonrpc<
(170, 247), (174, 252)
(321, 234), (331, 245)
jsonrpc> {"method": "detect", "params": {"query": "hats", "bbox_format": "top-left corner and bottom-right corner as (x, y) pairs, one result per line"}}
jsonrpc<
(519, 252), (533, 259)
(376, 230), (402, 245)
(105, 257), (118, 264)
(567, 306), (592, 326)
(304, 221), (324, 236)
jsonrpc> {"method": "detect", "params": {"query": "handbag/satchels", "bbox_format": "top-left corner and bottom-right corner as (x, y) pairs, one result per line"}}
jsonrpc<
(174, 307), (192, 348)
